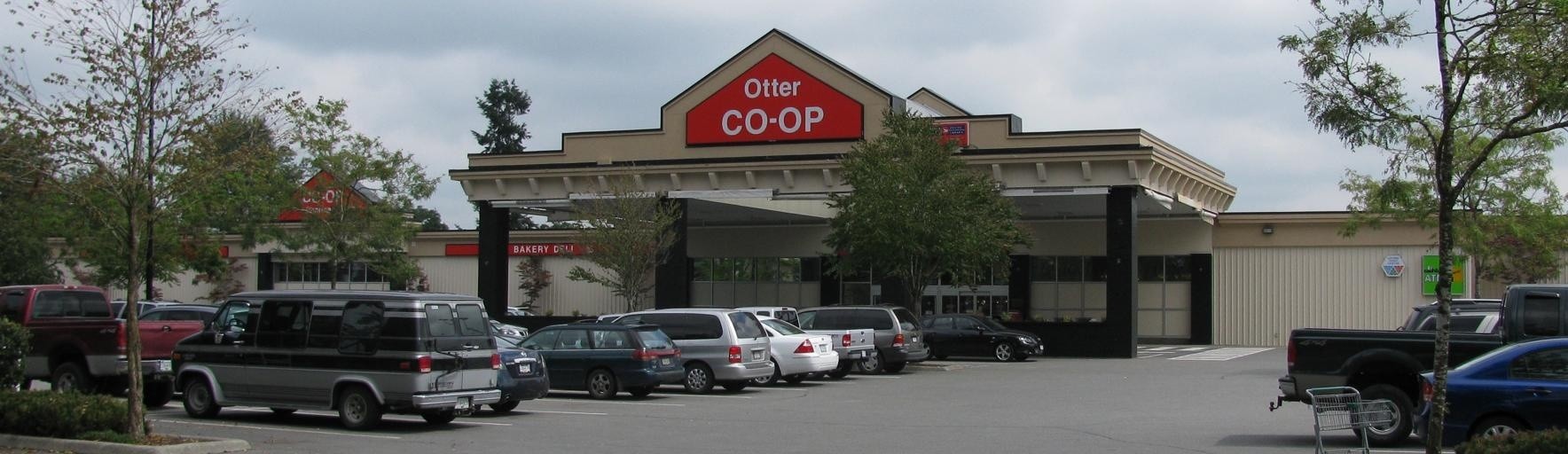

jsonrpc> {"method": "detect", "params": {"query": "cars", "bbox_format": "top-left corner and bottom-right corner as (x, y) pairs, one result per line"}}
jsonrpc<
(919, 314), (1044, 362)
(479, 304), (551, 413)
(1409, 336), (1568, 448)
(111, 299), (219, 321)
(702, 315), (839, 386)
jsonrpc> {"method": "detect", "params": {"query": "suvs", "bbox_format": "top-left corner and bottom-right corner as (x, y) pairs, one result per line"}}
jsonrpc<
(1394, 298), (1502, 335)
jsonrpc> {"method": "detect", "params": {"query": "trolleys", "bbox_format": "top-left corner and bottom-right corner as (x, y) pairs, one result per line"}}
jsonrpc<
(1305, 384), (1391, 454)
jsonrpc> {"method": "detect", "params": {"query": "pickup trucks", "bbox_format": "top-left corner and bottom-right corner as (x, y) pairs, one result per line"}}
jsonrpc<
(734, 306), (876, 380)
(0, 283), (205, 406)
(1269, 281), (1567, 447)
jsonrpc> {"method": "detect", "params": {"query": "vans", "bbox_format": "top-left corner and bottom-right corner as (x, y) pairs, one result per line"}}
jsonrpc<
(517, 323), (686, 400)
(796, 305), (930, 375)
(168, 289), (501, 430)
(611, 307), (776, 395)
